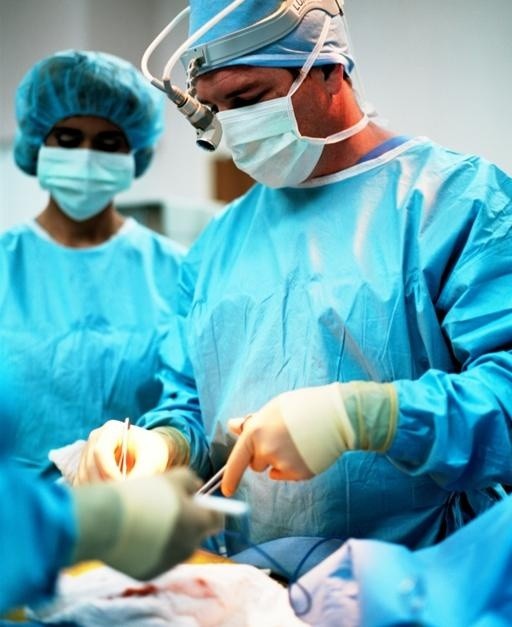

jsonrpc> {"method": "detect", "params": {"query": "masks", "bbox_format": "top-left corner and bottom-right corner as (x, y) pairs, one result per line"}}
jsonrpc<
(36, 145), (136, 223)
(215, 96), (327, 188)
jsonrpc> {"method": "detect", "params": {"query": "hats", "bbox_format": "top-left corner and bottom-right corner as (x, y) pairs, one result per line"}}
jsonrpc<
(188, 0), (355, 78)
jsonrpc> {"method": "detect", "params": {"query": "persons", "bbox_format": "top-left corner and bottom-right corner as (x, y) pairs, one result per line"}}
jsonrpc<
(0, 48), (189, 474)
(0, 462), (228, 615)
(70, 0), (512, 558)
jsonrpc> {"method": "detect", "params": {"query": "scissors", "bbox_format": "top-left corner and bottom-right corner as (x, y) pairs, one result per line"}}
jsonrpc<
(193, 414), (253, 496)
